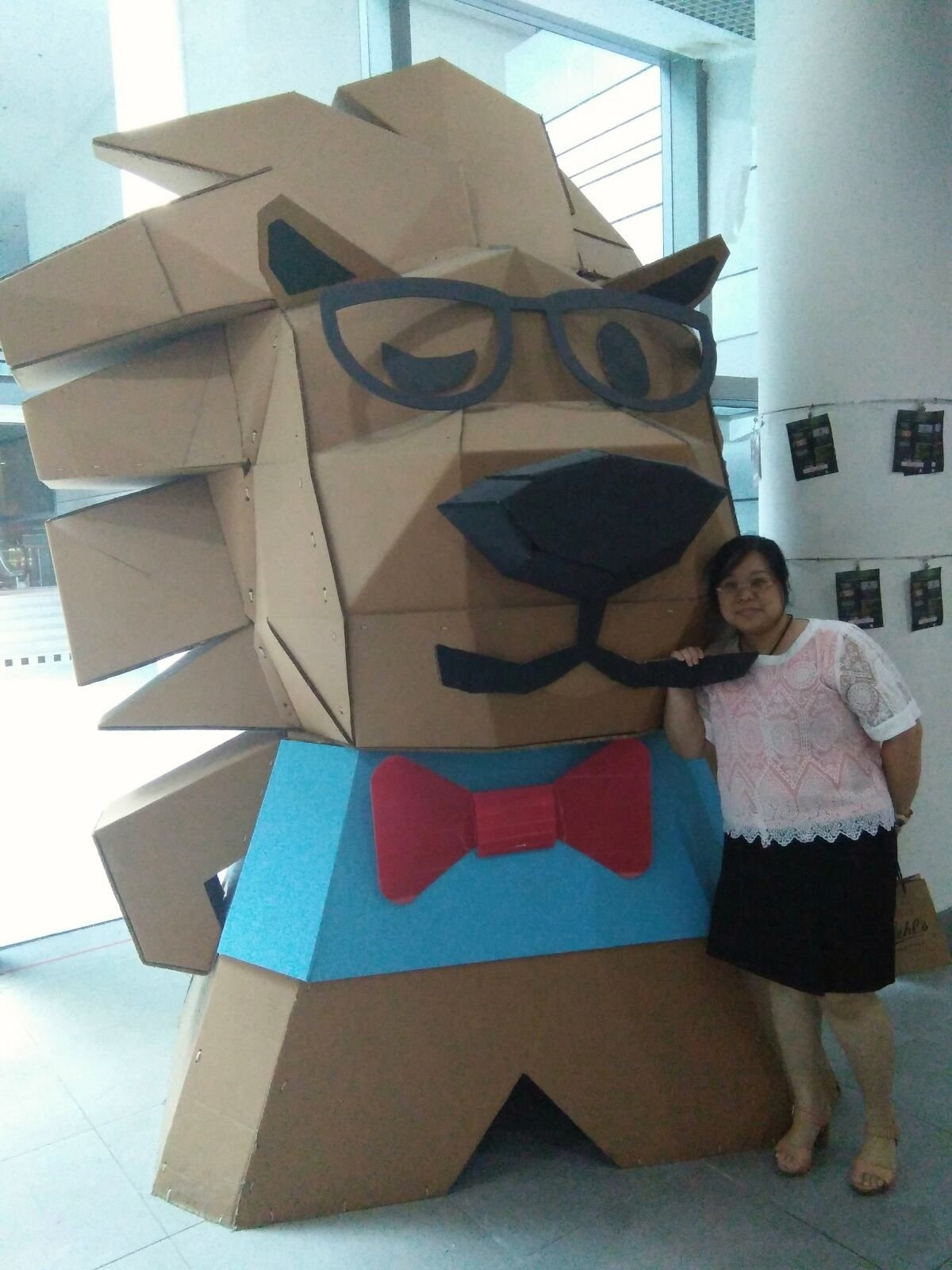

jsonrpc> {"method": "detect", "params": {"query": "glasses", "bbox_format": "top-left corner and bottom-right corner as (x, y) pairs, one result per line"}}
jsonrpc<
(716, 571), (777, 598)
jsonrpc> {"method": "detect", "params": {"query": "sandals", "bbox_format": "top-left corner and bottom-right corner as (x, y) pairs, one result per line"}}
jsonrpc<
(852, 1122), (901, 1193)
(776, 1101), (831, 1174)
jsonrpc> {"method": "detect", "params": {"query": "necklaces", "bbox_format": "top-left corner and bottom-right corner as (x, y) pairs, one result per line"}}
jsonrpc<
(737, 612), (793, 656)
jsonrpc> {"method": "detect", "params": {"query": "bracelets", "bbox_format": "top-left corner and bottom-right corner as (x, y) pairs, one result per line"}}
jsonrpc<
(893, 810), (914, 829)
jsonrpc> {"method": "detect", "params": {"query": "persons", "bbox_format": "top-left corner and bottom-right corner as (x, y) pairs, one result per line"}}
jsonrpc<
(662, 534), (930, 1198)
